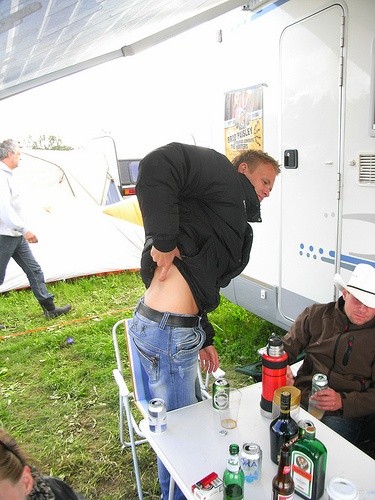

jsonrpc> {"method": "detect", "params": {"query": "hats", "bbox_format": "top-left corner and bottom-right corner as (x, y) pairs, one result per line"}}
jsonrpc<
(333, 263), (375, 308)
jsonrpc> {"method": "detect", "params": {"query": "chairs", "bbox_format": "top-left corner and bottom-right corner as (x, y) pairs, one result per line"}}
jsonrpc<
(113, 317), (226, 500)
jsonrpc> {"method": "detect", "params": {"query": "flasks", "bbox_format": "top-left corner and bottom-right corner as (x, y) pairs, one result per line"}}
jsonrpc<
(261, 336), (288, 413)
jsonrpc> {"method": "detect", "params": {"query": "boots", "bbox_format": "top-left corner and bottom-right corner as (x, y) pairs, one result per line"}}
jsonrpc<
(39, 298), (71, 321)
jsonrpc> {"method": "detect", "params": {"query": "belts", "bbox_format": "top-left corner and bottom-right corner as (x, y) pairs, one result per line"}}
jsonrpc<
(136, 302), (199, 328)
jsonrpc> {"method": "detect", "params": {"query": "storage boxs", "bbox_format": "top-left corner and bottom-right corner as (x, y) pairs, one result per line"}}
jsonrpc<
(191, 478), (224, 500)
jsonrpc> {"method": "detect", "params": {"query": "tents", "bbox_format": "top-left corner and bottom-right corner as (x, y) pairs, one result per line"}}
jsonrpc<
(0, 147), (147, 295)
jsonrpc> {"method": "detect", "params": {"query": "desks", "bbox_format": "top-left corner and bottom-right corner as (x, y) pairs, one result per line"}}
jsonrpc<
(139, 382), (375, 500)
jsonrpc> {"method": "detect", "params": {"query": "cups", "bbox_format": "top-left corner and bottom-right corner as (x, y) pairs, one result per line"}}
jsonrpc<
(217, 388), (242, 429)
(308, 394), (325, 419)
(325, 475), (361, 500)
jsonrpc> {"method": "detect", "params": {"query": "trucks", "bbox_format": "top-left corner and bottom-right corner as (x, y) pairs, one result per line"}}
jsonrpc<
(219, 0), (375, 334)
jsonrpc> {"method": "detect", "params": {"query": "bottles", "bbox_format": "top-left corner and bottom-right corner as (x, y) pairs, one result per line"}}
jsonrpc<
(272, 385), (301, 424)
(272, 447), (295, 500)
(222, 444), (245, 500)
(270, 391), (300, 465)
(288, 426), (327, 500)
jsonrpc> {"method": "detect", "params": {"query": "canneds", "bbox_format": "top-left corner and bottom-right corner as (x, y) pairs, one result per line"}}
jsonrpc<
(297, 420), (316, 439)
(147, 398), (167, 433)
(240, 442), (263, 483)
(211, 377), (230, 410)
(312, 373), (328, 395)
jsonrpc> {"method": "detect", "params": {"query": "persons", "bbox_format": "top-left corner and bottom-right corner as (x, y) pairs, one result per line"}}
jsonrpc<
(0, 138), (72, 320)
(280, 261), (375, 458)
(126, 142), (283, 499)
(0, 429), (78, 500)
